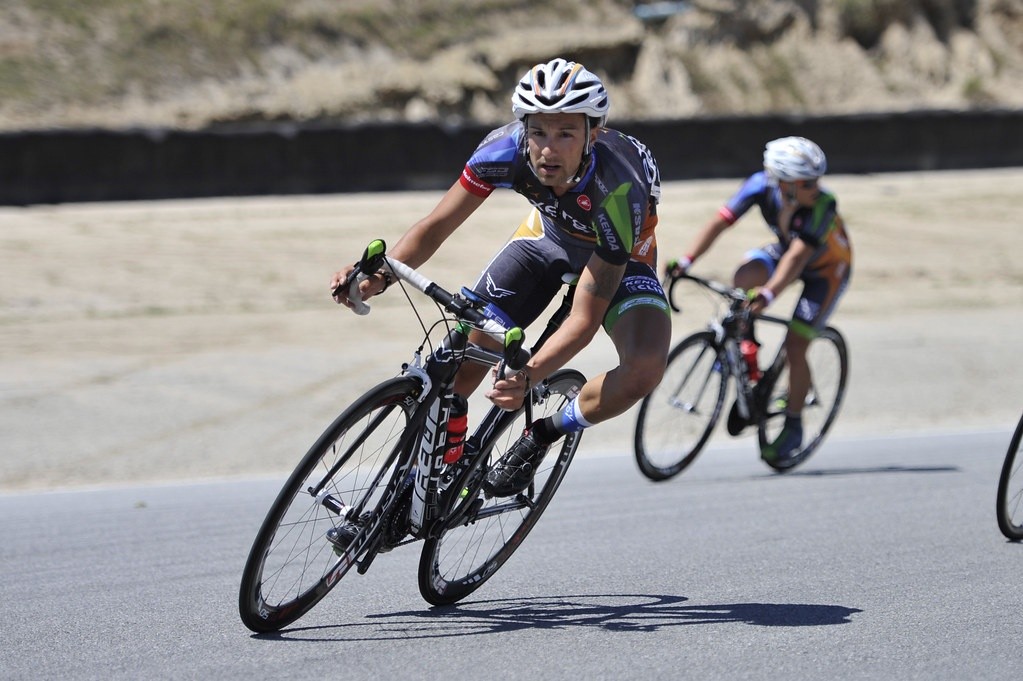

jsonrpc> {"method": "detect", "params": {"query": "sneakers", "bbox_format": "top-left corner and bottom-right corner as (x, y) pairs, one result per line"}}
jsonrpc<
(327, 510), (395, 553)
(762, 419), (802, 461)
(481, 419), (552, 497)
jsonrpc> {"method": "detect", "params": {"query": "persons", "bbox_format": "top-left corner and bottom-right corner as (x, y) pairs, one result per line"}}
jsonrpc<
(675, 136), (851, 459)
(324, 58), (671, 551)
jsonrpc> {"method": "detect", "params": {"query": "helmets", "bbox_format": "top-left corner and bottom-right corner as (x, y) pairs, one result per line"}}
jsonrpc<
(763, 136), (828, 182)
(511, 58), (609, 128)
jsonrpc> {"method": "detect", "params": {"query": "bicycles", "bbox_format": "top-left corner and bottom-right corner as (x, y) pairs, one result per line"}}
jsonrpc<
(239, 238), (587, 635)
(635, 257), (852, 481)
(996, 415), (1023, 541)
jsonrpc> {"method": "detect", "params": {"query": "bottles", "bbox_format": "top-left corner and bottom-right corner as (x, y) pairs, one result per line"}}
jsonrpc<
(441, 392), (468, 463)
(739, 339), (758, 381)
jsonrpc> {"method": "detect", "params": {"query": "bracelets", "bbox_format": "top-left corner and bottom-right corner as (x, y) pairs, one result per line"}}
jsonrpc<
(374, 270), (392, 295)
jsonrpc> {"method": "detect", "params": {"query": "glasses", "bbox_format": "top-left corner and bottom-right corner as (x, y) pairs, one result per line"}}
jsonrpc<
(792, 179), (818, 191)
